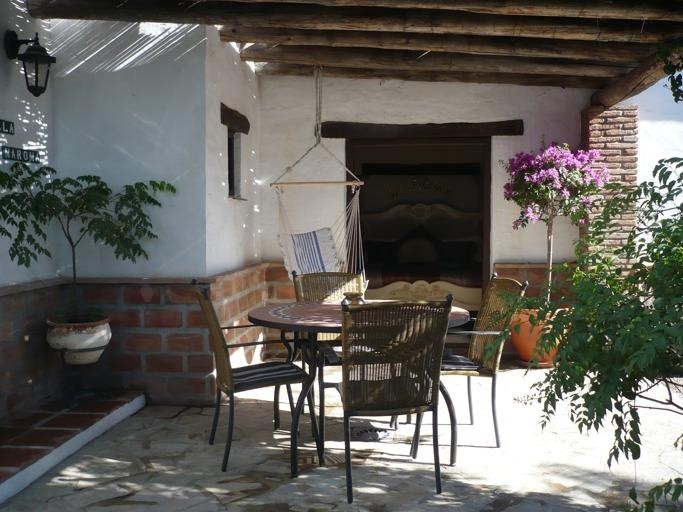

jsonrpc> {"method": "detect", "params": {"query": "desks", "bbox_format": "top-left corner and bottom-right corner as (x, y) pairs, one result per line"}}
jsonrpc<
(248, 303), (469, 467)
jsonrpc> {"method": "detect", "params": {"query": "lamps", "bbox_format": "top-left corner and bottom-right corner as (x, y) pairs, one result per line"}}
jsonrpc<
(3, 24), (59, 99)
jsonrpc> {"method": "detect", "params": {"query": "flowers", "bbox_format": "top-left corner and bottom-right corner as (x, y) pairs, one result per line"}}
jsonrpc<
(499, 130), (612, 308)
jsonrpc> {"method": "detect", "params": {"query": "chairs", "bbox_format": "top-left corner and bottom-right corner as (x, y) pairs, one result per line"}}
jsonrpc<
(336, 294), (454, 504)
(194, 284), (326, 471)
(293, 269), (367, 415)
(407, 272), (529, 460)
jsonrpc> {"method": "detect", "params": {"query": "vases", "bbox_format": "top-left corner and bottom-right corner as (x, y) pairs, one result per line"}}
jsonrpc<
(509, 309), (578, 369)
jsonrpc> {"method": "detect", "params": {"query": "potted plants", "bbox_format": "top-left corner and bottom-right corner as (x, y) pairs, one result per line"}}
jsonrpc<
(0, 159), (180, 366)
